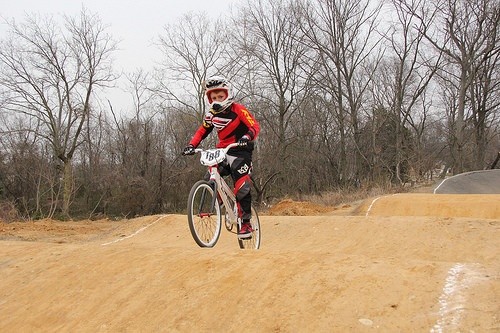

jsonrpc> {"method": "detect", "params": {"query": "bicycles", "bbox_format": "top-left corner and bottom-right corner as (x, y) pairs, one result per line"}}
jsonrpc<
(176, 139), (271, 251)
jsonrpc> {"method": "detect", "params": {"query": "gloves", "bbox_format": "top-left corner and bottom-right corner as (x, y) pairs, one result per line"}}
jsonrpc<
(183, 144), (196, 155)
(237, 134), (249, 147)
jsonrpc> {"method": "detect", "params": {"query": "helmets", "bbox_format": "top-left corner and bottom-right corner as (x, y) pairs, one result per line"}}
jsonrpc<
(204, 74), (235, 114)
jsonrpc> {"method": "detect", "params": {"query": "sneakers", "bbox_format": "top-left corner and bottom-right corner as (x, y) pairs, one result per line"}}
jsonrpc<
(237, 222), (254, 239)
(215, 191), (225, 208)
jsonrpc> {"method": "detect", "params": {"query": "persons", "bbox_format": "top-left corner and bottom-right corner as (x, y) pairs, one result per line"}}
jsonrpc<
(183, 76), (260, 239)
